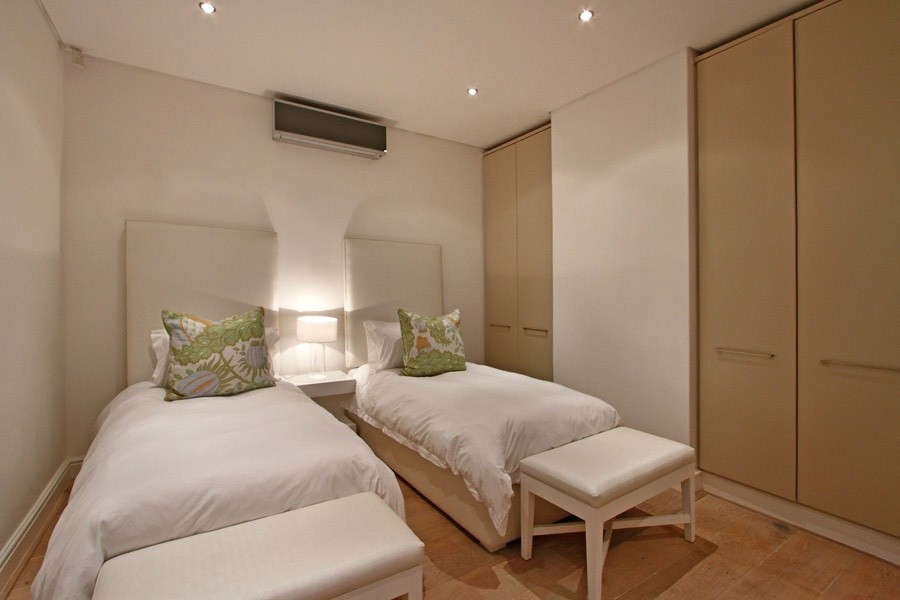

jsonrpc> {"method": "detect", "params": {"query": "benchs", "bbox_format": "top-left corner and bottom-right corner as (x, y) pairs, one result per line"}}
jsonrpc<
(519, 426), (696, 600)
(91, 491), (426, 600)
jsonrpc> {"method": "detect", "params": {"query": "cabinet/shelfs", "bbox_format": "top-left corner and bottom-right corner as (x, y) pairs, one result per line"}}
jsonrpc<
(693, 0), (900, 567)
(481, 121), (555, 384)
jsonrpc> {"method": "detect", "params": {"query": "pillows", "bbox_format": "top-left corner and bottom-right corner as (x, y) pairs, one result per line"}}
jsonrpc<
(363, 320), (405, 378)
(149, 308), (280, 402)
(396, 307), (467, 379)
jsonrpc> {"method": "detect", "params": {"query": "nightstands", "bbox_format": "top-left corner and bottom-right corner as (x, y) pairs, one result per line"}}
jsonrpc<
(284, 370), (357, 432)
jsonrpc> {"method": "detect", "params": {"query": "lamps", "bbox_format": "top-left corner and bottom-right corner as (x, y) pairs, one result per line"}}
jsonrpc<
(295, 314), (338, 377)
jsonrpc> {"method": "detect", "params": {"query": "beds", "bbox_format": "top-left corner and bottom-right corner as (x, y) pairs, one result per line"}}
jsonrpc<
(343, 235), (636, 554)
(30, 218), (406, 600)
